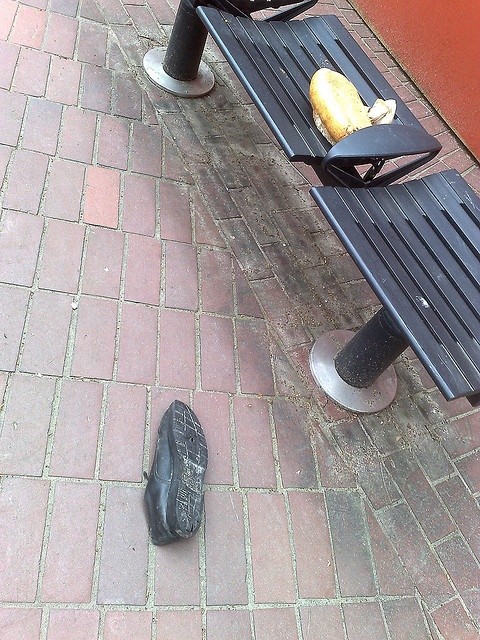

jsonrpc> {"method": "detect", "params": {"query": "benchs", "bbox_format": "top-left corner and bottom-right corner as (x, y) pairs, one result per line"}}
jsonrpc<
(143, 0), (480, 413)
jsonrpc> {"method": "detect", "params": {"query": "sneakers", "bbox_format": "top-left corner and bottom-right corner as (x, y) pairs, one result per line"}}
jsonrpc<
(143, 400), (208, 545)
